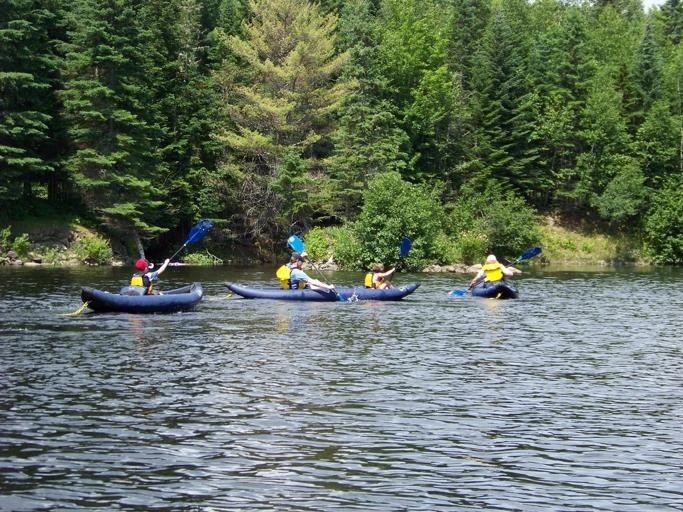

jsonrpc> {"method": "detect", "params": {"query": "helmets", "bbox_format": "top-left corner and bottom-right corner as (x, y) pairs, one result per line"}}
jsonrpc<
(294, 255), (306, 262)
(135, 258), (148, 271)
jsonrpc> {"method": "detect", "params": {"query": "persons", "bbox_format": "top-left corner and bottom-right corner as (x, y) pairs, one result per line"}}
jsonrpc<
(364, 261), (397, 289)
(130, 257), (169, 295)
(470, 254), (513, 288)
(276, 252), (335, 290)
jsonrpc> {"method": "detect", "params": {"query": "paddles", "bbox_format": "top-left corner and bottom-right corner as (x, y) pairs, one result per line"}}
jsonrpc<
(390, 237), (412, 282)
(169, 220), (214, 260)
(286, 235), (347, 301)
(449, 247), (542, 296)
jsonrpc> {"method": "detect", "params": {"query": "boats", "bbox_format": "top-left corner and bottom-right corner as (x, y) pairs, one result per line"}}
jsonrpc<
(223, 281), (420, 301)
(471, 282), (518, 298)
(79, 281), (203, 316)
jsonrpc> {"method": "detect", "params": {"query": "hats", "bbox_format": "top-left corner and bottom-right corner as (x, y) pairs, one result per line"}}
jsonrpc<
(487, 254), (497, 263)
(146, 261), (154, 269)
